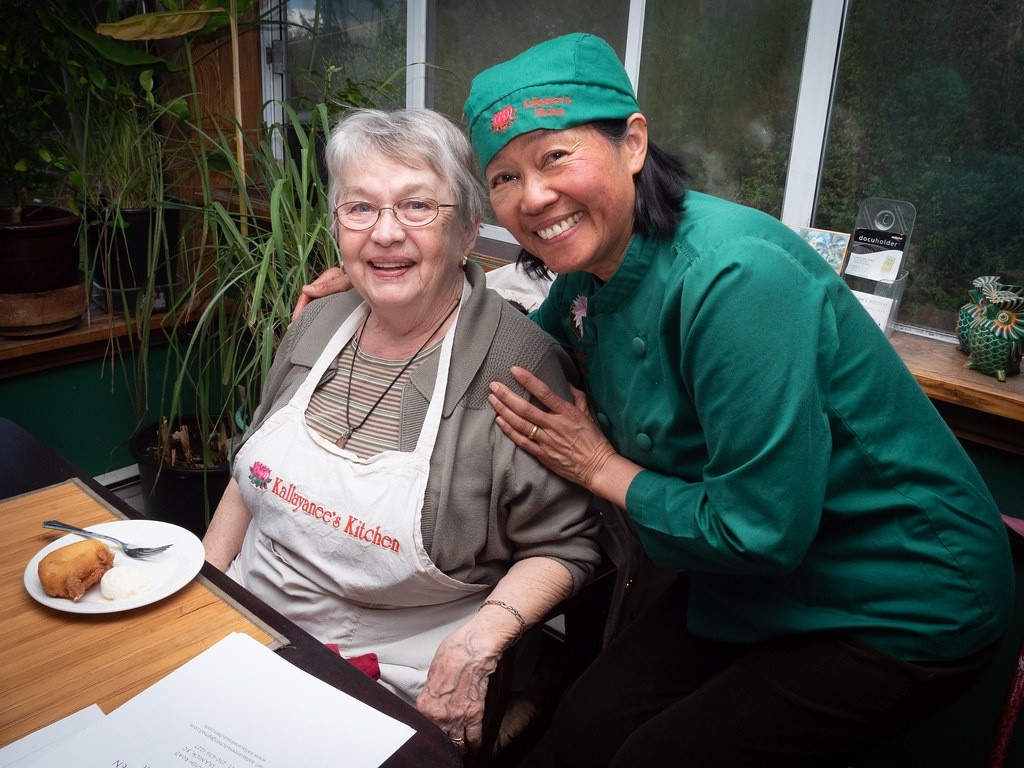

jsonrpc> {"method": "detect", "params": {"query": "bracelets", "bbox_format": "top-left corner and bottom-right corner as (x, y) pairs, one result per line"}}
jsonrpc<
(479, 600), (526, 647)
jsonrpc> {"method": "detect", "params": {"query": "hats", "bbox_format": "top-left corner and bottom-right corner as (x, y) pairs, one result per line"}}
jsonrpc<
(464, 32), (641, 175)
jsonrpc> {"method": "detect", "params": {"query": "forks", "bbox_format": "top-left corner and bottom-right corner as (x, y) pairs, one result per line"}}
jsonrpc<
(41, 521), (173, 559)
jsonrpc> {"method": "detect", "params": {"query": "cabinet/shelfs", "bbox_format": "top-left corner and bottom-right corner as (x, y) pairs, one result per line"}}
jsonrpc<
(197, 185), (1024, 458)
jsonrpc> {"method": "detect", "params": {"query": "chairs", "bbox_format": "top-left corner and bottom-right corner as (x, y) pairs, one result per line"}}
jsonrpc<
(477, 561), (614, 768)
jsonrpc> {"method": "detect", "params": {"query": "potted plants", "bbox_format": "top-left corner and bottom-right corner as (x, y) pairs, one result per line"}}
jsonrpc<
(0, 0), (466, 541)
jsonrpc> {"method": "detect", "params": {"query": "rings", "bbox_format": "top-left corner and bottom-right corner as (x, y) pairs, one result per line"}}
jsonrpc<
(528, 426), (538, 440)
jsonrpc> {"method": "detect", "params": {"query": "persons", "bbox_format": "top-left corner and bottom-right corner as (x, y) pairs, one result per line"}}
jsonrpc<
(290, 30), (1024, 768)
(202, 102), (608, 748)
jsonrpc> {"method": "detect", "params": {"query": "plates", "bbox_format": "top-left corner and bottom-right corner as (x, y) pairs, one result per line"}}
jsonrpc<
(24, 520), (204, 614)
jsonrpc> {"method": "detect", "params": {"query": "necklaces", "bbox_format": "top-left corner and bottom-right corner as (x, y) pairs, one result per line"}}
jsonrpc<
(335, 293), (464, 449)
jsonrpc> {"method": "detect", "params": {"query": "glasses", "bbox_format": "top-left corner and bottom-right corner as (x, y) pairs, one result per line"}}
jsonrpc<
(333, 197), (461, 230)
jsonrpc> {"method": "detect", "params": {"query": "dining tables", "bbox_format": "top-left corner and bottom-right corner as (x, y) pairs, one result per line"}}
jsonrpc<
(0, 417), (468, 767)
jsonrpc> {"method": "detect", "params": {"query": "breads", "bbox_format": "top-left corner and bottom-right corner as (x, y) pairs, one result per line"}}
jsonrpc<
(37, 540), (116, 602)
